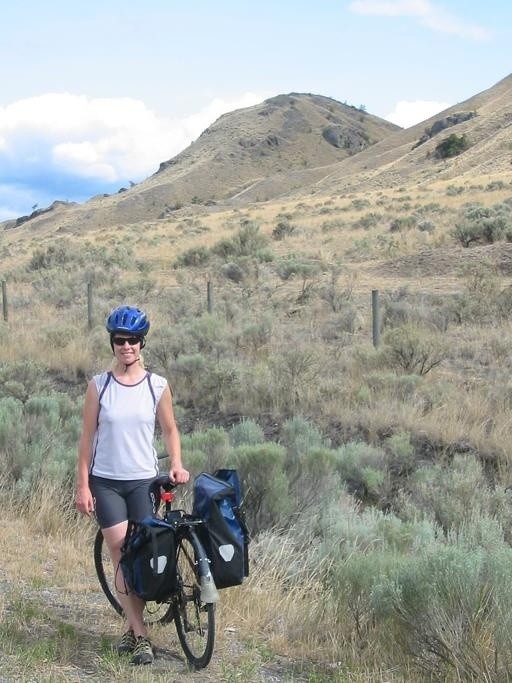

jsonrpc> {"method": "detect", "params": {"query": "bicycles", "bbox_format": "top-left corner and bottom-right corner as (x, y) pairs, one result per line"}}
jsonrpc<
(94, 452), (249, 669)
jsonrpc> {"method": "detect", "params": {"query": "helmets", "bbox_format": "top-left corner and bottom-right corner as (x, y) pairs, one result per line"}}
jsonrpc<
(106, 304), (150, 336)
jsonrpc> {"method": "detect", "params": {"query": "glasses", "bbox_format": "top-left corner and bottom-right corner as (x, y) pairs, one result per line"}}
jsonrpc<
(110, 336), (141, 346)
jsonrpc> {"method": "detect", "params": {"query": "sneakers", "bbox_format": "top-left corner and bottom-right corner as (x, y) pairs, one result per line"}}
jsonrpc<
(116, 630), (136, 654)
(132, 637), (155, 665)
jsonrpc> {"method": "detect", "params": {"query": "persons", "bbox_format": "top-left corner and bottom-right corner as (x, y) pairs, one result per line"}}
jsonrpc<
(75, 305), (191, 664)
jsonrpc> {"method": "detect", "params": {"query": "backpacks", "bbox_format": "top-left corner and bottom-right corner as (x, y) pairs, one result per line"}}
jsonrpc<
(193, 466), (252, 590)
(114, 516), (183, 605)
